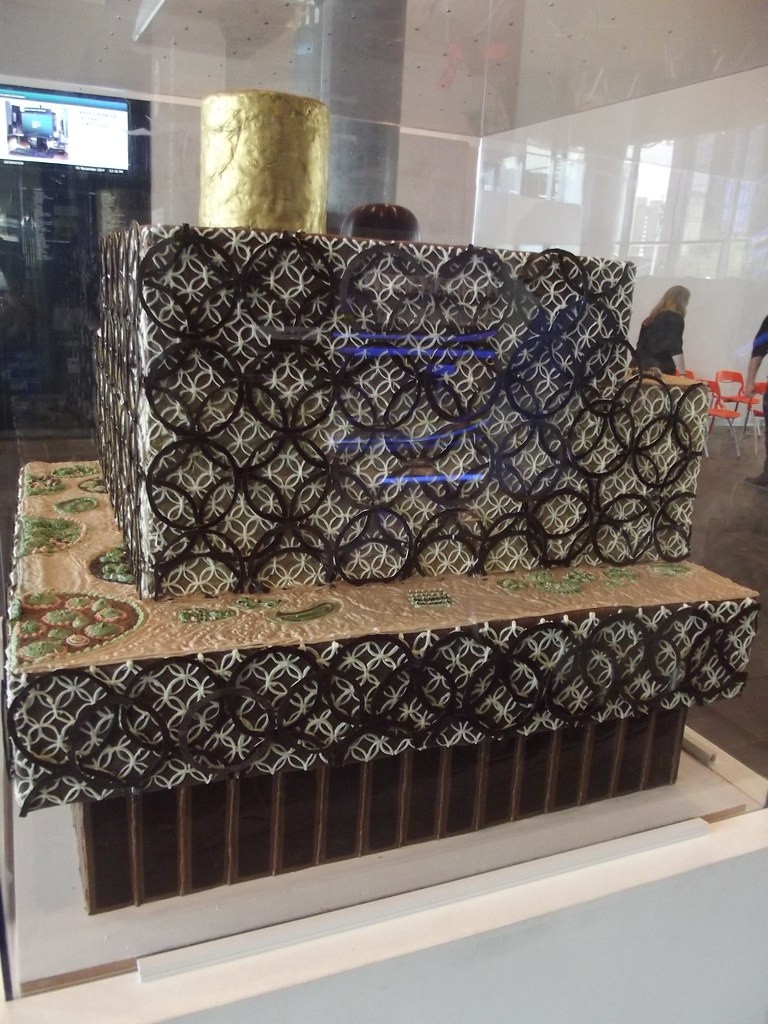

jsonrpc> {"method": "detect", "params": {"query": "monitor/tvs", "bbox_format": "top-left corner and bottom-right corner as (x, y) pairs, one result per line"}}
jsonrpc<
(4, 101), (68, 138)
(0, 84), (133, 181)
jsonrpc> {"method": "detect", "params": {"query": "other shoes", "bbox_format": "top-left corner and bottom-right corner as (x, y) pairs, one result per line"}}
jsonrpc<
(744, 474), (768, 490)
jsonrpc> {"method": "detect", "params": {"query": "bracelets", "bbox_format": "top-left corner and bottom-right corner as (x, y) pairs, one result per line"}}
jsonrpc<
(680, 373), (686, 375)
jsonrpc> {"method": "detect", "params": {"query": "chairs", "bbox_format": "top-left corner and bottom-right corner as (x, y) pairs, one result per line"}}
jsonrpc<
(676, 370), (768, 459)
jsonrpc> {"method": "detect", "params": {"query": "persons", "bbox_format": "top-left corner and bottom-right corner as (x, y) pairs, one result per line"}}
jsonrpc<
(629, 286), (690, 379)
(743, 314), (768, 490)
